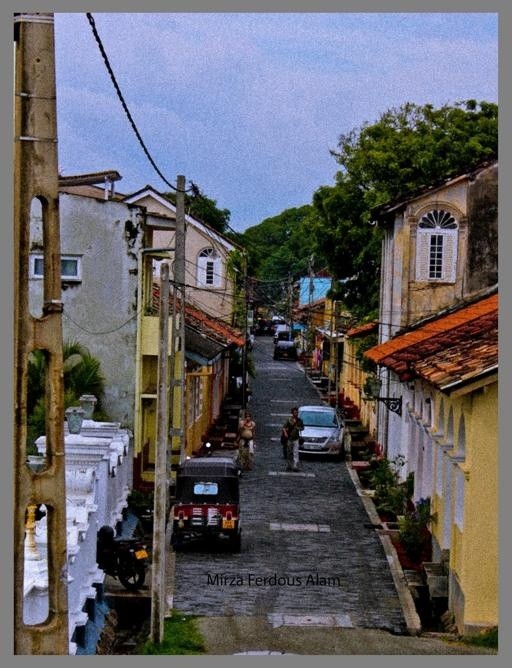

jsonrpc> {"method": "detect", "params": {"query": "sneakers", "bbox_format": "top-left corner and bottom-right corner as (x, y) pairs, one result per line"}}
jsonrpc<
(286, 466), (301, 472)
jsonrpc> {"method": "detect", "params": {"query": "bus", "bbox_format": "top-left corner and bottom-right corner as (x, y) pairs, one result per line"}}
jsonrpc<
(169, 456), (243, 556)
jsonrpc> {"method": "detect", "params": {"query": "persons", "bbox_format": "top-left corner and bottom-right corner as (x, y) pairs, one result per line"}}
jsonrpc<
(239, 412), (256, 471)
(282, 408), (304, 472)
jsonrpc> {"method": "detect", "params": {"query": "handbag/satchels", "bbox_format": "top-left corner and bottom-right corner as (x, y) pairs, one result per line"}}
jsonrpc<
(280, 435), (289, 444)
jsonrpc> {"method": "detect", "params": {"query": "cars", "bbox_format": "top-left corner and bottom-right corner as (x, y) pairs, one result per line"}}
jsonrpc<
(273, 340), (298, 362)
(274, 324), (295, 344)
(296, 405), (345, 457)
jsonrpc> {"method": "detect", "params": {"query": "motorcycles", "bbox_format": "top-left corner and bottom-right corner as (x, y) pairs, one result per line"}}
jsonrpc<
(97, 526), (150, 590)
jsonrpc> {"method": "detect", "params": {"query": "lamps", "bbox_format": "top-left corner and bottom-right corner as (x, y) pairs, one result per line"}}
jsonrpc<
(363, 383), (402, 418)
